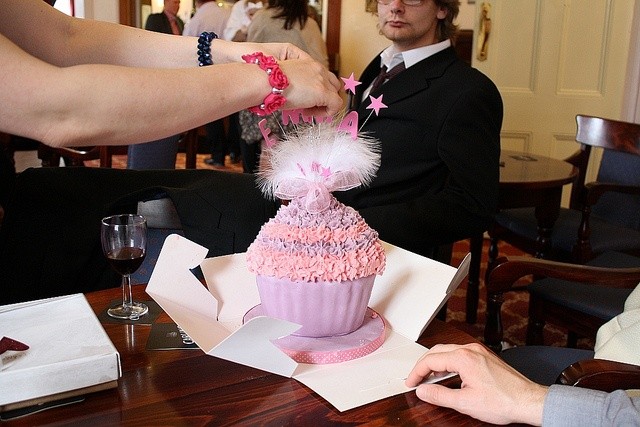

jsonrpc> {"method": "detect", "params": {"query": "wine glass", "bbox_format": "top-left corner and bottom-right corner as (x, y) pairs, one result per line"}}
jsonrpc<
(101, 213), (148, 319)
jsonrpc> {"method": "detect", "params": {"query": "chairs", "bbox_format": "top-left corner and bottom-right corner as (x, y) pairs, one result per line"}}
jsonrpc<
(483, 261), (640, 390)
(526, 249), (640, 345)
(464, 115), (640, 325)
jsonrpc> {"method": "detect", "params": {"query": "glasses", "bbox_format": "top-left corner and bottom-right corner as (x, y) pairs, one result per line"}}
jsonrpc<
(377, 0), (427, 7)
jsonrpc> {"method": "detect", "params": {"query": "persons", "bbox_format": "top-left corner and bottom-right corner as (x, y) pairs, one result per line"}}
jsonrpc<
(226, 0), (268, 43)
(0, 0), (341, 148)
(346, 0), (503, 324)
(184, 0), (242, 167)
(243, 0), (330, 151)
(146, 0), (184, 38)
(404, 343), (639, 426)
(494, 284), (640, 383)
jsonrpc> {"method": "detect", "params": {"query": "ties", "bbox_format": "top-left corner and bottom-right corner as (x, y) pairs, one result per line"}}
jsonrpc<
(371, 64), (403, 92)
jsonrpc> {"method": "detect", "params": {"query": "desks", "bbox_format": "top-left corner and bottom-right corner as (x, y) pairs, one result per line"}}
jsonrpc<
(460, 151), (580, 322)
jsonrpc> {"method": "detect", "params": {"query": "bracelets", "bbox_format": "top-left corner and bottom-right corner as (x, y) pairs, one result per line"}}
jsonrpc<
(195, 32), (217, 67)
(241, 51), (289, 117)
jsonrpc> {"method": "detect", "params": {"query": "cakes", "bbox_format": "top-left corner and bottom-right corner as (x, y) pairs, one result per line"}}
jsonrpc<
(243, 72), (390, 340)
